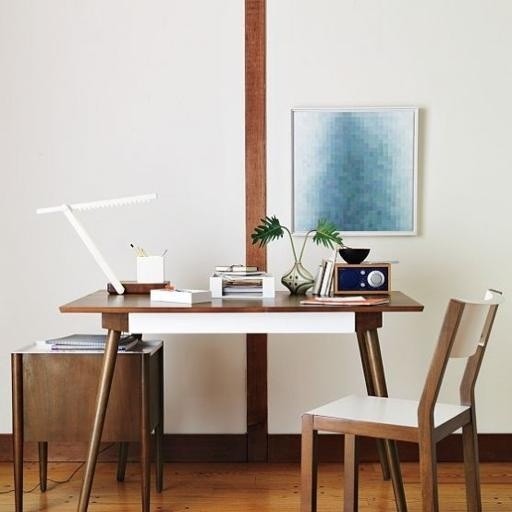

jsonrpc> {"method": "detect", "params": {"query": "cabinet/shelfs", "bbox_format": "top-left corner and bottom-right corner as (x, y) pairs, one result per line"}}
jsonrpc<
(11, 342), (162, 512)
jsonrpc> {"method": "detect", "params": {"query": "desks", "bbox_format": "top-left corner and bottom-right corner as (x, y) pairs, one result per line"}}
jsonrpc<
(60, 291), (424, 512)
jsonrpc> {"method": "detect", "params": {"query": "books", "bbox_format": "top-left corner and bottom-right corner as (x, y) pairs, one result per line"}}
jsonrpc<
(214, 265), (268, 293)
(45, 333), (140, 350)
(300, 296), (390, 306)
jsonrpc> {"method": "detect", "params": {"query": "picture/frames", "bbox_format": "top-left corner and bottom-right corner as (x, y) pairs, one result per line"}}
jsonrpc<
(289, 107), (417, 238)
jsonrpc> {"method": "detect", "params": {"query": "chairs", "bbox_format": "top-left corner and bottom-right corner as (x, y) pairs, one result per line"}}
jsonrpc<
(301, 289), (503, 512)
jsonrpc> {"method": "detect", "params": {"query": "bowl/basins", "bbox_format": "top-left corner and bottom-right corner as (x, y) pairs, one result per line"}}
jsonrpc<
(338, 248), (371, 264)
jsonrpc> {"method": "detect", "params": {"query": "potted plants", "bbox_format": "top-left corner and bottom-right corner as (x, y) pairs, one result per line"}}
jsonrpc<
(250, 215), (344, 296)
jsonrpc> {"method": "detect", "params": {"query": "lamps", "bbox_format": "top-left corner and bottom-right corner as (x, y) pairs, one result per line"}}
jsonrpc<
(37, 193), (170, 294)
